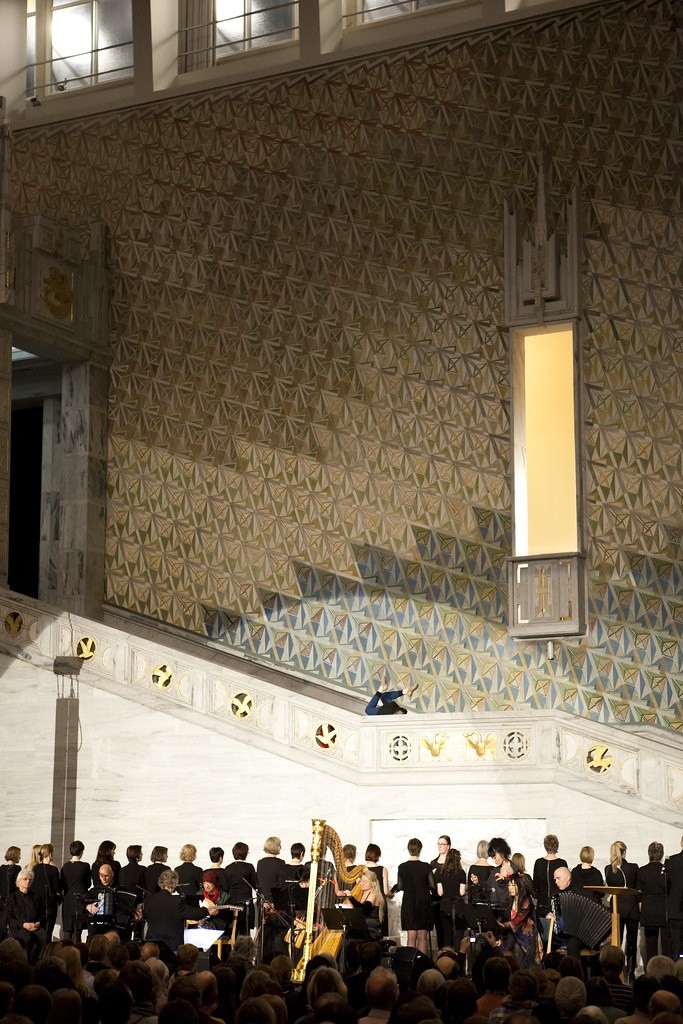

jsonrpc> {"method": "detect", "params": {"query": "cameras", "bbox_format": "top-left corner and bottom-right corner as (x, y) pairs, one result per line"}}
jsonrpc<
(476, 933), (487, 939)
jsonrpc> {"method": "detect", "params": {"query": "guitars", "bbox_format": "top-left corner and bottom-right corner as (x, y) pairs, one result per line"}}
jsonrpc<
(185, 898), (245, 925)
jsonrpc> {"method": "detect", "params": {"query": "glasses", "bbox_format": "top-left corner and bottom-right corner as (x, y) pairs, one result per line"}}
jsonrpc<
(436, 843), (448, 846)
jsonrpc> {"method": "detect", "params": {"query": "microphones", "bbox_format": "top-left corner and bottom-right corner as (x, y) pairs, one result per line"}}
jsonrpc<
(480, 888), (496, 892)
(617, 865), (626, 887)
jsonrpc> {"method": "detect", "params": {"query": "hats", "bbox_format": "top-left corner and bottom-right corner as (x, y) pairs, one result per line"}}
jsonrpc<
(202, 871), (217, 885)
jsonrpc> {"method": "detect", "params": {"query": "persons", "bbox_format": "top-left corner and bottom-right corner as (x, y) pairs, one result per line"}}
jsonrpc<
(365, 676), (418, 716)
(1, 834), (683, 1024)
